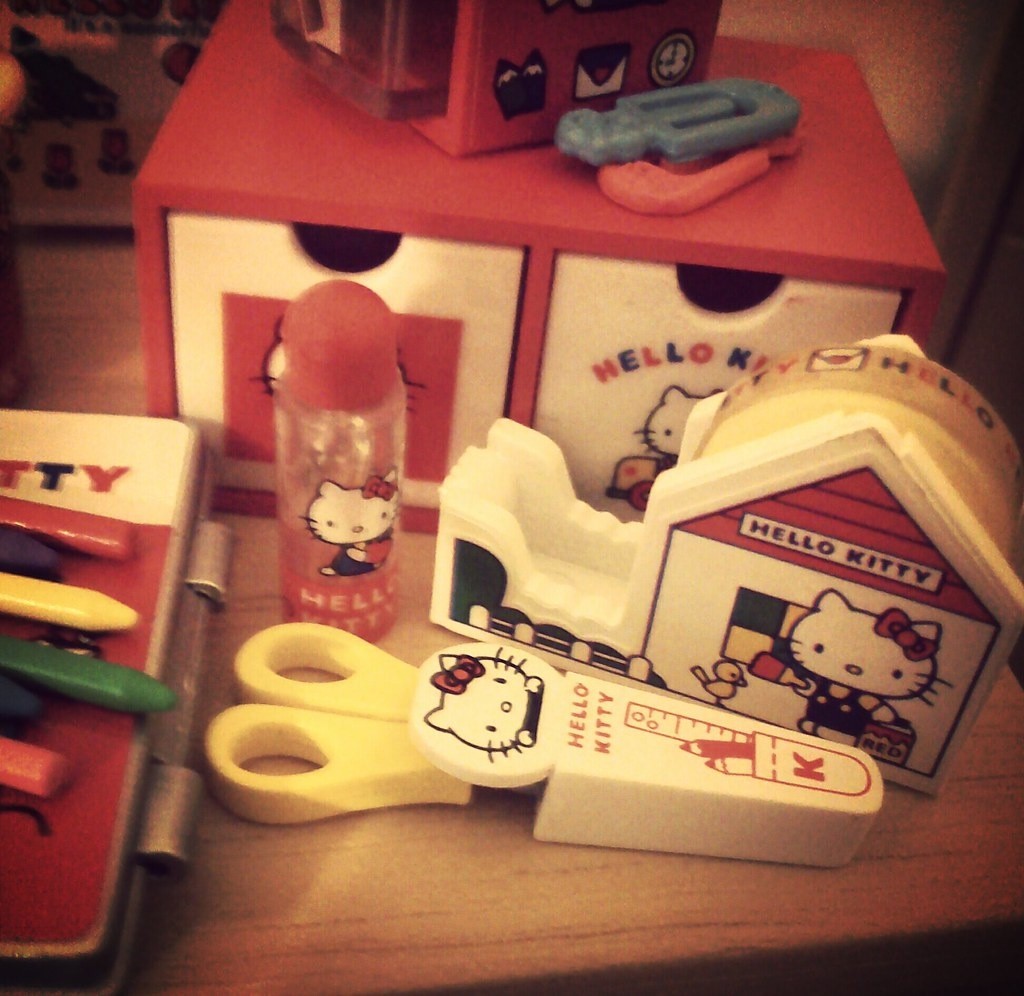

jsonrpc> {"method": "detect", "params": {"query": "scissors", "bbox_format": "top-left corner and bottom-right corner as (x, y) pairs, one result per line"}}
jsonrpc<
(198, 621), (550, 826)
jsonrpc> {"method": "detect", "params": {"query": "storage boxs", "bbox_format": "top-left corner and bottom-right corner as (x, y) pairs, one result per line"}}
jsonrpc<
(267, 0), (725, 158)
(130, 0), (949, 534)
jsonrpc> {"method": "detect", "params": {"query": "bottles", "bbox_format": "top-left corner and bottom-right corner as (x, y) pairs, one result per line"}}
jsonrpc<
(267, 280), (407, 651)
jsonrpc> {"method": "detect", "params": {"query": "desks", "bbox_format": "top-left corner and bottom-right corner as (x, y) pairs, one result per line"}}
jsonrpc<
(0, 232), (1024, 996)
